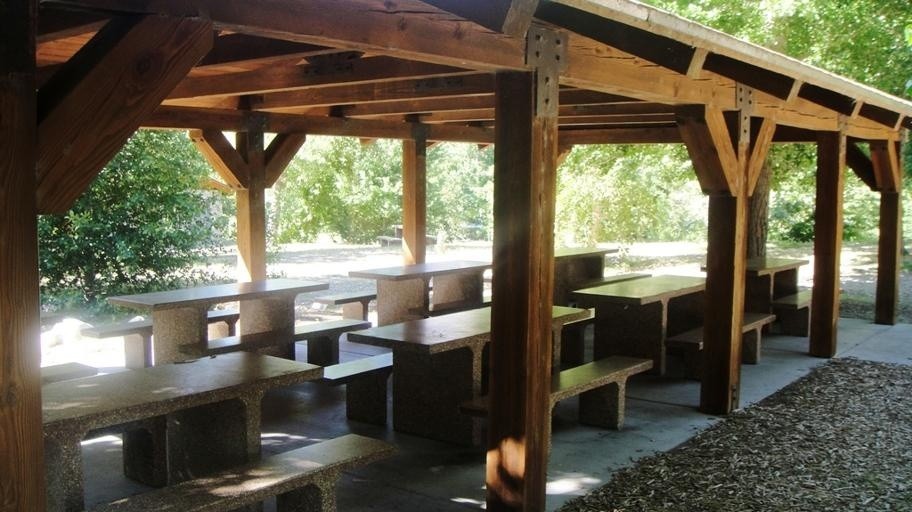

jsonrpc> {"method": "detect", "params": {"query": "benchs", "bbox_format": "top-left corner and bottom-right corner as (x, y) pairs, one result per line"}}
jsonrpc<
(315, 251), (813, 381)
(323, 305), (654, 445)
(41, 363), (396, 511)
(81, 276), (372, 363)
